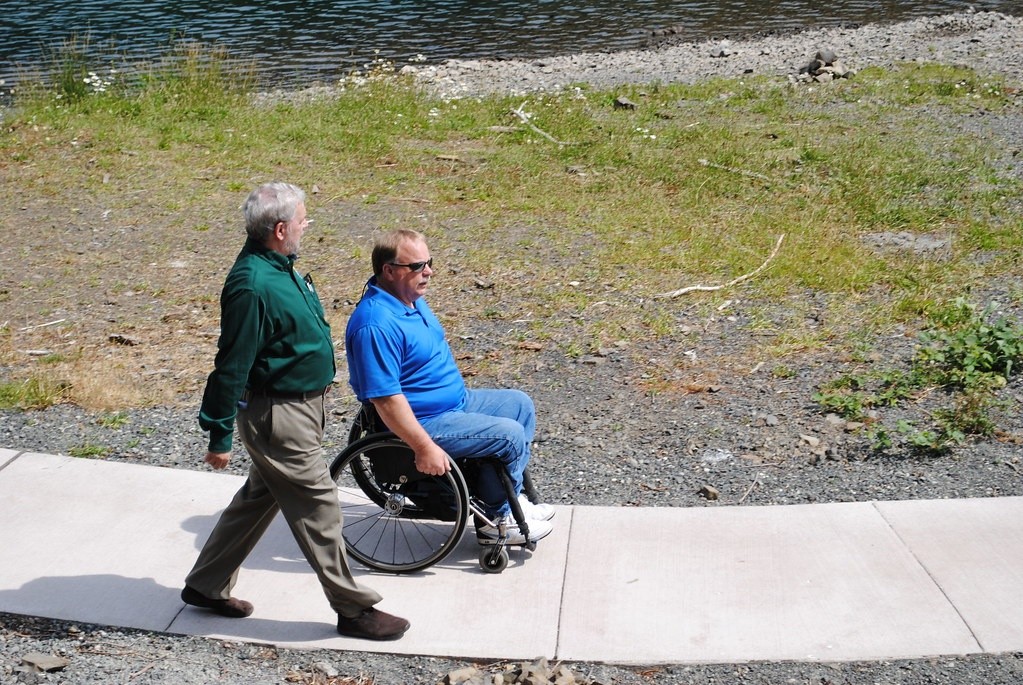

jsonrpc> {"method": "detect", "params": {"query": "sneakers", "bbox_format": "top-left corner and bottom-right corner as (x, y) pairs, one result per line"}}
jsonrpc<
(506, 494), (556, 521)
(475, 515), (553, 545)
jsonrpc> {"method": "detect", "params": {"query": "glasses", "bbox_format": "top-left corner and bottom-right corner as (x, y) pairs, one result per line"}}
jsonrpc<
(387, 257), (433, 272)
(304, 272), (313, 286)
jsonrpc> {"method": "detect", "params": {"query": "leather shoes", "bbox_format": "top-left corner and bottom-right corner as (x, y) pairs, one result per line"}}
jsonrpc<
(181, 585), (254, 618)
(337, 608), (410, 638)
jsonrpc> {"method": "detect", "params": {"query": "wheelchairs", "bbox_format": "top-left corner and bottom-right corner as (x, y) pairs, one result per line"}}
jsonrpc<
(329, 403), (541, 574)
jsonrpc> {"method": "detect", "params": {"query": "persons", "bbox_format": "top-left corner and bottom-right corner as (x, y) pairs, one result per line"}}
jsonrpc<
(345, 229), (556, 546)
(180, 182), (410, 640)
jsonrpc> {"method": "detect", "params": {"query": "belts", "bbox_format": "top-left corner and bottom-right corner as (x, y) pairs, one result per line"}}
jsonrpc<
(245, 384), (332, 399)
